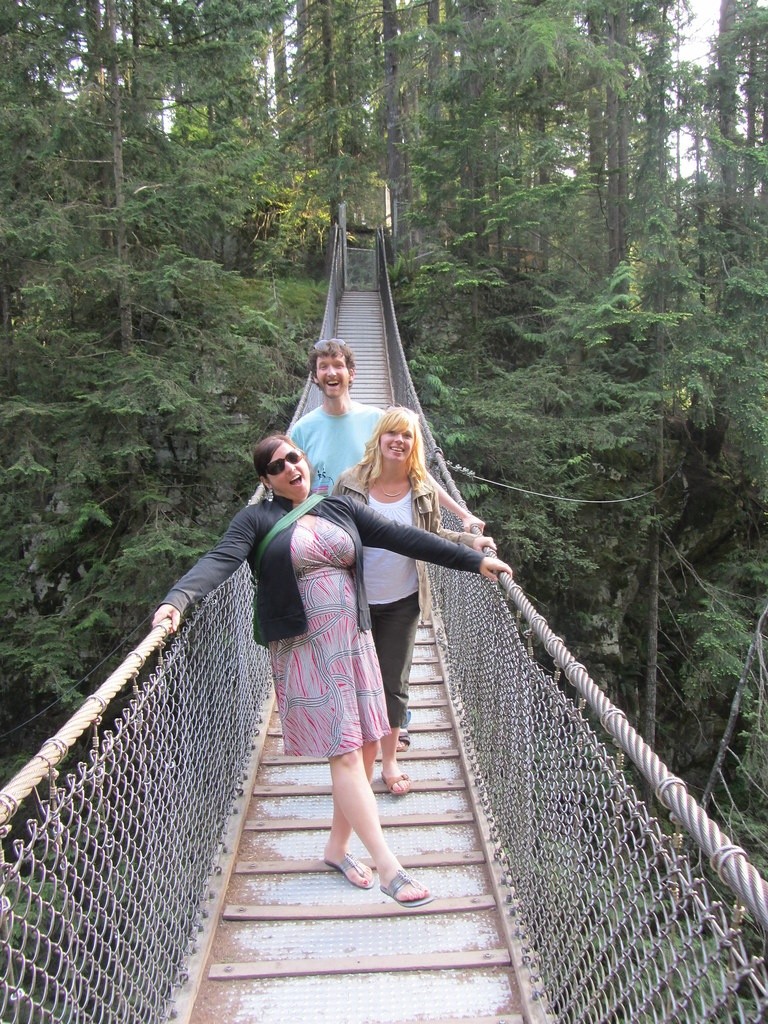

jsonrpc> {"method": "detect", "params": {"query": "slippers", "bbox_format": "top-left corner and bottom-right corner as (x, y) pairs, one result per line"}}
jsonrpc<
(394, 731), (410, 752)
(380, 868), (434, 907)
(380, 771), (411, 796)
(324, 853), (374, 888)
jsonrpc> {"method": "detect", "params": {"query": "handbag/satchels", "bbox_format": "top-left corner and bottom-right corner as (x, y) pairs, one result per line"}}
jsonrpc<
(253, 576), (268, 650)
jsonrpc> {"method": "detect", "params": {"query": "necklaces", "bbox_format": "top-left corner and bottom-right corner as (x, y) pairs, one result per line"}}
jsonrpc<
(378, 477), (409, 496)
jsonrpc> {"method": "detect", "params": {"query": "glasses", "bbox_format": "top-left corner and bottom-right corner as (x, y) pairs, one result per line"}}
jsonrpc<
(314, 339), (346, 350)
(265, 449), (304, 475)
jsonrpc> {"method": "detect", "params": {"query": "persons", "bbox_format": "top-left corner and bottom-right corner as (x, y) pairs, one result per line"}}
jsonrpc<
(332, 407), (496, 798)
(152, 435), (514, 909)
(288, 340), (485, 754)
